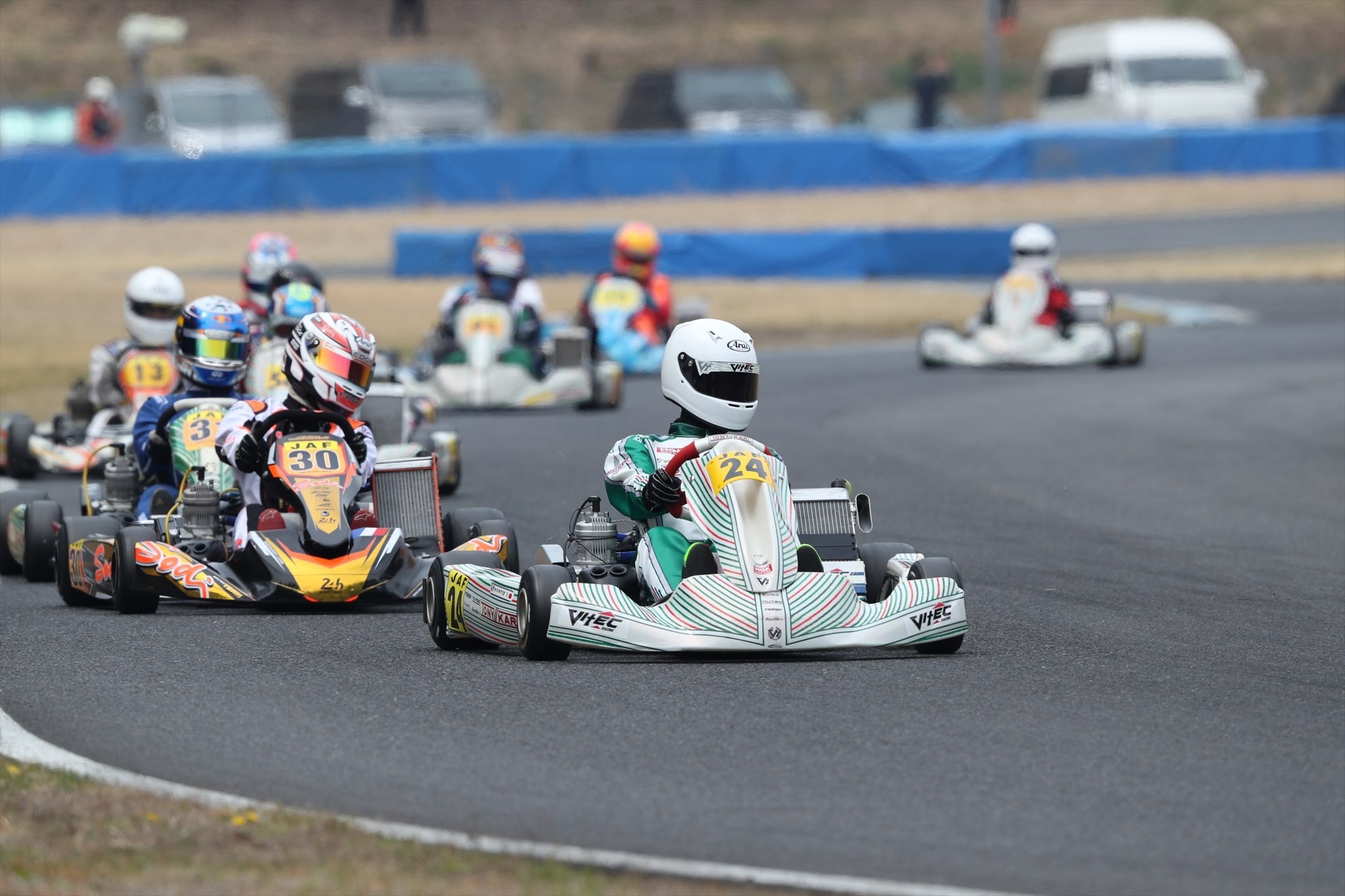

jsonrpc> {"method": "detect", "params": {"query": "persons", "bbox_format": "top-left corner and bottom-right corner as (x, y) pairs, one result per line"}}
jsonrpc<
(427, 231), (555, 376)
(602, 315), (824, 601)
(975, 222), (1076, 341)
(905, 41), (958, 126)
(92, 267), (190, 458)
(211, 315), (377, 528)
(131, 294), (255, 515)
(576, 225), (671, 353)
(390, 0), (431, 36)
(234, 231), (331, 400)
(75, 64), (121, 143)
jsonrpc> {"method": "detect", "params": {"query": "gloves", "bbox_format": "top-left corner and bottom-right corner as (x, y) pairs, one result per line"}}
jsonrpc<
(342, 432), (368, 464)
(237, 421), (269, 469)
(148, 429), (171, 468)
(641, 467), (683, 513)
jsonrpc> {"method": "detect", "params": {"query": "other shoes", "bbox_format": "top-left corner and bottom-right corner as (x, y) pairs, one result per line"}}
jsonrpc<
(797, 544), (824, 573)
(258, 509), (286, 532)
(681, 542), (718, 579)
(108, 415), (123, 425)
(152, 488), (177, 516)
(352, 509), (377, 529)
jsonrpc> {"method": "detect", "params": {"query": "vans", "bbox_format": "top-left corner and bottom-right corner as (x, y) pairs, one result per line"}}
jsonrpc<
(1035, 17), (1268, 133)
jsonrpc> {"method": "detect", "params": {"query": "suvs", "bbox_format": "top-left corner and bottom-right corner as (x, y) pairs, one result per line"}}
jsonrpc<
(292, 58), (506, 141)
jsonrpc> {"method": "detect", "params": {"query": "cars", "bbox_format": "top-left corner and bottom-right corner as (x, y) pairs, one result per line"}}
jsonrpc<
(616, 61), (835, 136)
(115, 74), (295, 155)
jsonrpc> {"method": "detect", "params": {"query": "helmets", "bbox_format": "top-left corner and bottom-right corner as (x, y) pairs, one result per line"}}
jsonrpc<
(1009, 221), (1061, 270)
(269, 262), (322, 293)
(269, 283), (326, 336)
(661, 318), (760, 432)
(120, 265), (185, 346)
(280, 310), (377, 419)
(474, 226), (524, 276)
(246, 234), (297, 288)
(616, 223), (660, 284)
(173, 295), (252, 388)
